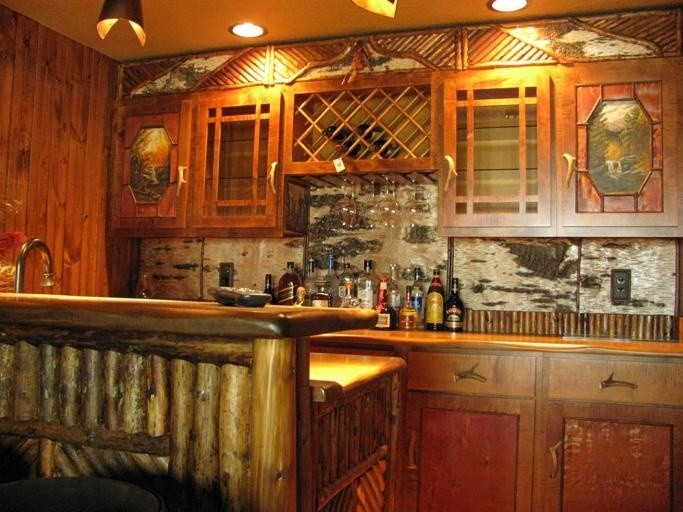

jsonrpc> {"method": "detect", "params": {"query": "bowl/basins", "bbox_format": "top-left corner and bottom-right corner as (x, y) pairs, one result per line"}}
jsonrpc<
(208, 287), (272, 307)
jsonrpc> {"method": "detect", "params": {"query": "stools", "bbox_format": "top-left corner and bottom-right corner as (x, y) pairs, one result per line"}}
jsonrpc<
(0, 478), (170, 511)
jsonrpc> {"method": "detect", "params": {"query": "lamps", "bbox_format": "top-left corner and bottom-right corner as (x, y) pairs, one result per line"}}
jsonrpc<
(352, 0), (399, 18)
(95, 0), (149, 48)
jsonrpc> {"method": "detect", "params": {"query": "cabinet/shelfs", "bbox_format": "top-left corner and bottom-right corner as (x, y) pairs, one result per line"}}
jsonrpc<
(109, 87), (193, 239)
(309, 336), (399, 358)
(531, 340), (682, 512)
(186, 81), (310, 242)
(282, 65), (441, 176)
(554, 54), (682, 240)
(434, 59), (557, 238)
(394, 338), (545, 512)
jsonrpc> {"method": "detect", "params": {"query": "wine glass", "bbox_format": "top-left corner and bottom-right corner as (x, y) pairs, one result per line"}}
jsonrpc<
(333, 176), (433, 230)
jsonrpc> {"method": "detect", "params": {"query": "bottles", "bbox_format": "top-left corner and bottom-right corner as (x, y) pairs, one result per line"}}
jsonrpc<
(260, 251), (337, 308)
(444, 275), (464, 335)
(337, 256), (443, 330)
(323, 124), (400, 161)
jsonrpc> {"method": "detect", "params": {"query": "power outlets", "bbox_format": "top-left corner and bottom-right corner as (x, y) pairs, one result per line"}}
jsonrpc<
(610, 269), (630, 306)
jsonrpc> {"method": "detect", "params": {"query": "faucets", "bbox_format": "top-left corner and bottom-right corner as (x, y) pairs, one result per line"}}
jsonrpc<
(15, 238), (55, 293)
